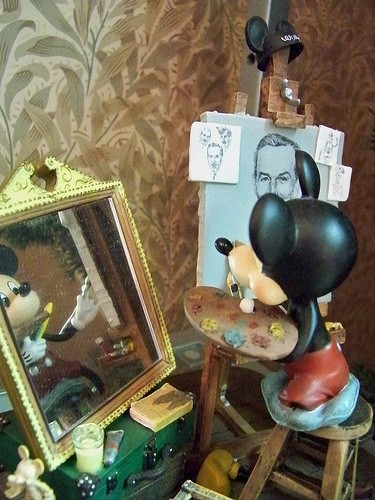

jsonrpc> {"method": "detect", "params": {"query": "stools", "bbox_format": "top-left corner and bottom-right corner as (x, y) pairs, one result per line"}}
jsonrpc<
(237, 396), (373, 500)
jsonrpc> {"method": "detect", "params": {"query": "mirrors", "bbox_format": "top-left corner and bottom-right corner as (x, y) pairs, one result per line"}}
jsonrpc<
(0, 157), (177, 471)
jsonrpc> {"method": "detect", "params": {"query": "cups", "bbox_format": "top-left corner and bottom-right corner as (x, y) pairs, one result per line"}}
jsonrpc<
(72, 423), (104, 474)
